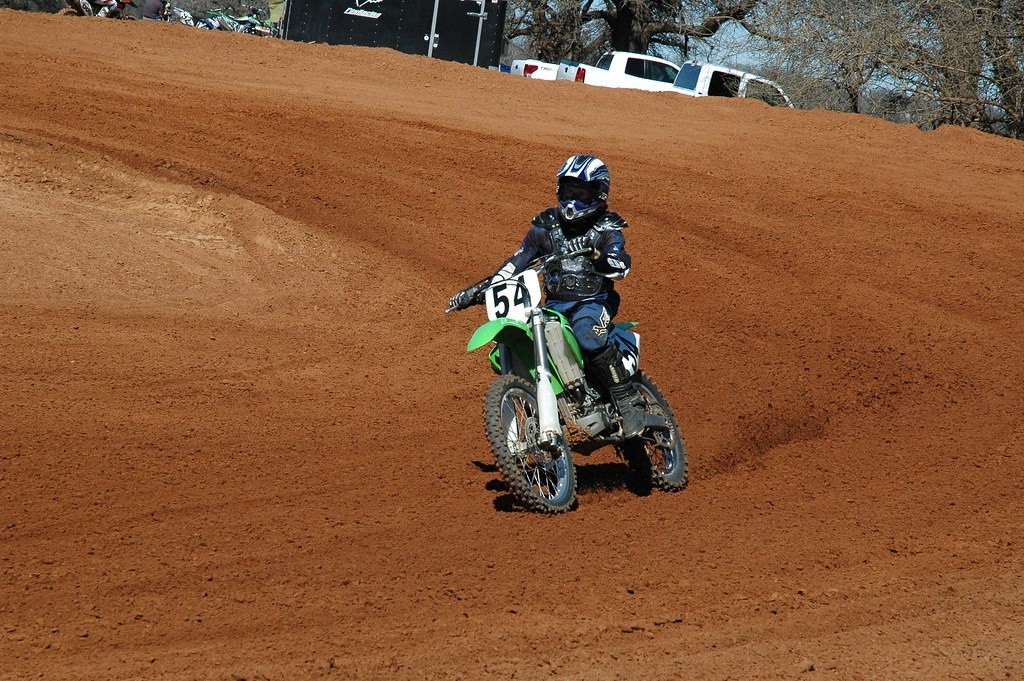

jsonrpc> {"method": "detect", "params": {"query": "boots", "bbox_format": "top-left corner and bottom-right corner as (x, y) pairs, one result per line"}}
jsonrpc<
(588, 343), (648, 440)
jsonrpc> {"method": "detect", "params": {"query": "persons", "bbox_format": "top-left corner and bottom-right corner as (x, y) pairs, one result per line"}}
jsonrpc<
(143, 0), (170, 22)
(451, 154), (648, 439)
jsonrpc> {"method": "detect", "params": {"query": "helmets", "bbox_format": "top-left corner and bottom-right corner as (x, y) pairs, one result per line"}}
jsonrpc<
(555, 154), (611, 222)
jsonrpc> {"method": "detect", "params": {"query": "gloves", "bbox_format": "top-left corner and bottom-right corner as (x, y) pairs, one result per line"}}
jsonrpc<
(449, 291), (469, 312)
(566, 236), (596, 261)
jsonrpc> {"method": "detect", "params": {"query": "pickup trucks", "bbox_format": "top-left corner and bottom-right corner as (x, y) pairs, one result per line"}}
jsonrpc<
(511, 51), (795, 109)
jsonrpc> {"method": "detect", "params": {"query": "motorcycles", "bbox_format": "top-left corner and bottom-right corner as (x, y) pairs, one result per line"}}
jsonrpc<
(169, 6), (283, 37)
(445, 247), (689, 516)
